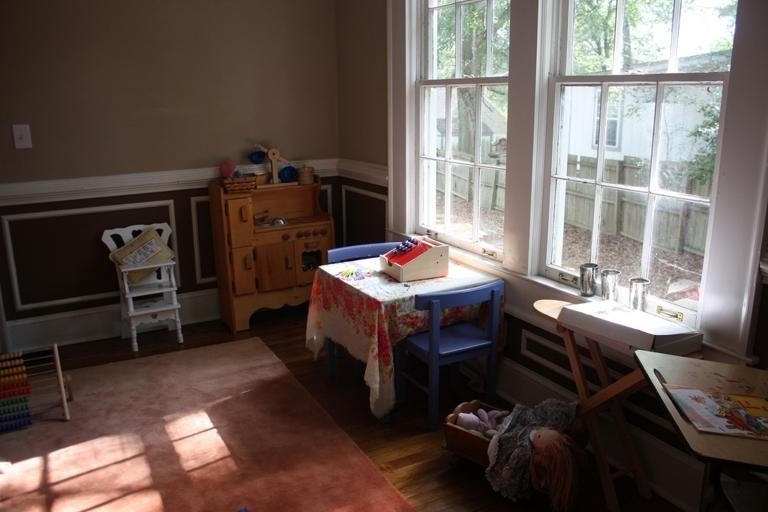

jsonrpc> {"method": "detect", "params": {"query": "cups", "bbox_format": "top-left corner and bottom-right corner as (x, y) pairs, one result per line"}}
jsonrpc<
(296, 164), (314, 185)
(579, 260), (650, 313)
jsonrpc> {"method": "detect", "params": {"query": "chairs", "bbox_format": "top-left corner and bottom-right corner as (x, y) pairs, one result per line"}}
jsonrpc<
(324, 239), (401, 264)
(400, 278), (506, 430)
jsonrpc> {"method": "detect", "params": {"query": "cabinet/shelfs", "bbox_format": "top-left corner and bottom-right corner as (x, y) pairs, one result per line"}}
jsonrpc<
(205, 176), (334, 336)
(101, 222), (182, 352)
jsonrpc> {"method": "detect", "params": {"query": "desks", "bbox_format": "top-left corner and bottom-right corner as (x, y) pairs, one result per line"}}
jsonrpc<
(531, 298), (709, 512)
(304, 255), (507, 419)
(631, 340), (767, 512)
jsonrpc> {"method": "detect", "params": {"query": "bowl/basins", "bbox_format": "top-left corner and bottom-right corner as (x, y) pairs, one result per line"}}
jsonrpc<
(238, 172), (272, 186)
(271, 218), (285, 225)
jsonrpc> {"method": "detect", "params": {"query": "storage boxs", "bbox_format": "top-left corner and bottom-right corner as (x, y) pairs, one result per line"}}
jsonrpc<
(556, 298), (702, 367)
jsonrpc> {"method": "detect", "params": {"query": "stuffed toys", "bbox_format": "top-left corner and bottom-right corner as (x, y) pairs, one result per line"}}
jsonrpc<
(456, 397), (579, 512)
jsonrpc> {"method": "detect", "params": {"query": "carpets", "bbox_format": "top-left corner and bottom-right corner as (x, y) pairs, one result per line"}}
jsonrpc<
(0, 334), (415, 512)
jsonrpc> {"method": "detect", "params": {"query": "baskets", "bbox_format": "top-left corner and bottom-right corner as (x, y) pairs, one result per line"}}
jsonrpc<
(223, 173), (256, 193)
